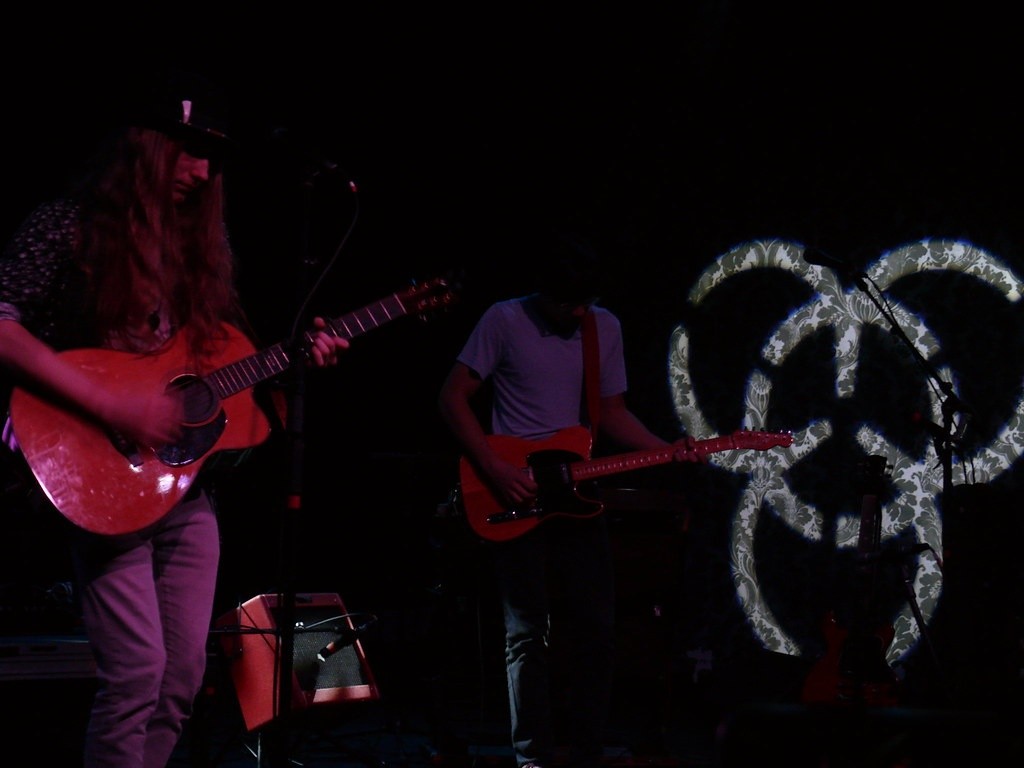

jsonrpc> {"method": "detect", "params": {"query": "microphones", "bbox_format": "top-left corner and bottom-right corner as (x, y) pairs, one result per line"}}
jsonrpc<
(863, 543), (929, 564)
(319, 160), (361, 193)
(321, 620), (376, 658)
(804, 247), (868, 278)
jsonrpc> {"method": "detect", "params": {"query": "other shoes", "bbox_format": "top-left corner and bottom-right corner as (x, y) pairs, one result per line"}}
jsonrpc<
(519, 762), (540, 768)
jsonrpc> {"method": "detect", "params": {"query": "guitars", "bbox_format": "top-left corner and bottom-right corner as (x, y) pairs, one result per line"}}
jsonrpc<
(4, 272), (466, 557)
(456, 425), (794, 543)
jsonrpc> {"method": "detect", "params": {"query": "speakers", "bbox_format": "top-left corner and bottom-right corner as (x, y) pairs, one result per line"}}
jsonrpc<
(217, 594), (380, 732)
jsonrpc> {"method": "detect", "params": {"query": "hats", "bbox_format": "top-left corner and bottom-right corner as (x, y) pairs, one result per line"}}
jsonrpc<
(143, 80), (243, 159)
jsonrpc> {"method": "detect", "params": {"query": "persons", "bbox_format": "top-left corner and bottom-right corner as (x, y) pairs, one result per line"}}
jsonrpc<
(443, 247), (708, 768)
(0, 96), (349, 768)
